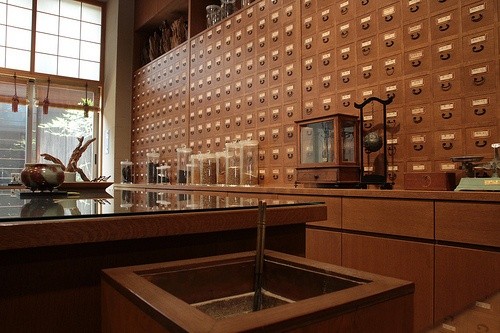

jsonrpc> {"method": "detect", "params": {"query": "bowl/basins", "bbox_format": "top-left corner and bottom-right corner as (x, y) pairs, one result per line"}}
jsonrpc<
(21, 163), (65, 192)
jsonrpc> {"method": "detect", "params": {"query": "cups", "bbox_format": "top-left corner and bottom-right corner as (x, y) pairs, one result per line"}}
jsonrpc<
(146, 153), (171, 185)
(120, 161), (132, 184)
(177, 141), (259, 187)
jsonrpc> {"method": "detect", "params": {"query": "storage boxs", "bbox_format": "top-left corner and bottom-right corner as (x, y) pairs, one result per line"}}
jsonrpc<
(403, 171), (456, 191)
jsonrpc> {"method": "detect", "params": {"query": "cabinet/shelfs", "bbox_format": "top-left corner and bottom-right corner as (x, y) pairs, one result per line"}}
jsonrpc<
(131, 0), (500, 333)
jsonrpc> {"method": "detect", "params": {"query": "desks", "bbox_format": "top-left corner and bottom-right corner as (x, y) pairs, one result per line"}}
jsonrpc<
(0, 188), (328, 333)
